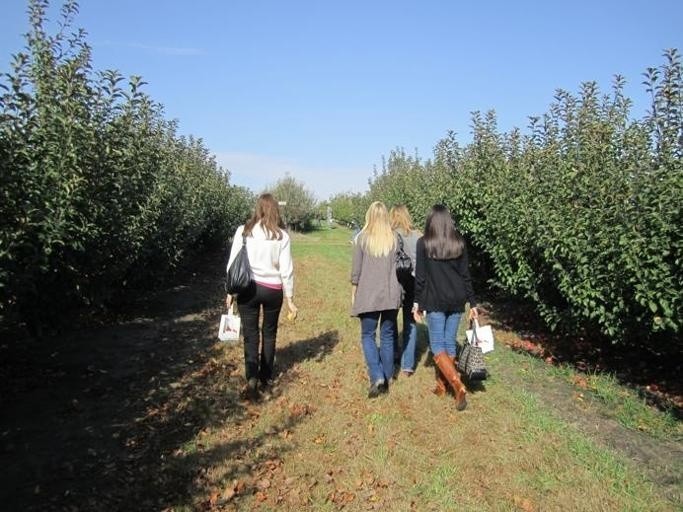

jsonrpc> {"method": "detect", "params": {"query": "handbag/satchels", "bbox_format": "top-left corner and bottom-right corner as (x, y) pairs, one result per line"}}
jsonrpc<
(457, 319), (487, 381)
(226, 232), (251, 293)
(465, 319), (495, 355)
(396, 253), (413, 286)
(217, 304), (243, 342)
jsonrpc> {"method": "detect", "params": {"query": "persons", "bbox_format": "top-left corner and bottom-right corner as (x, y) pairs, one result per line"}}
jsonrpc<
(411, 204), (478, 410)
(350, 201), (406, 397)
(388, 203), (423, 377)
(225, 194), (297, 398)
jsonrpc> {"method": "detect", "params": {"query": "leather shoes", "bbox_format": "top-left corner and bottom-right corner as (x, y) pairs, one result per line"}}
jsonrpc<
(367, 379), (384, 398)
(242, 378), (258, 395)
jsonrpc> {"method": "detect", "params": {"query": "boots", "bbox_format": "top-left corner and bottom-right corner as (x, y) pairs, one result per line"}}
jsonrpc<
(434, 355), (456, 399)
(432, 351), (468, 411)
